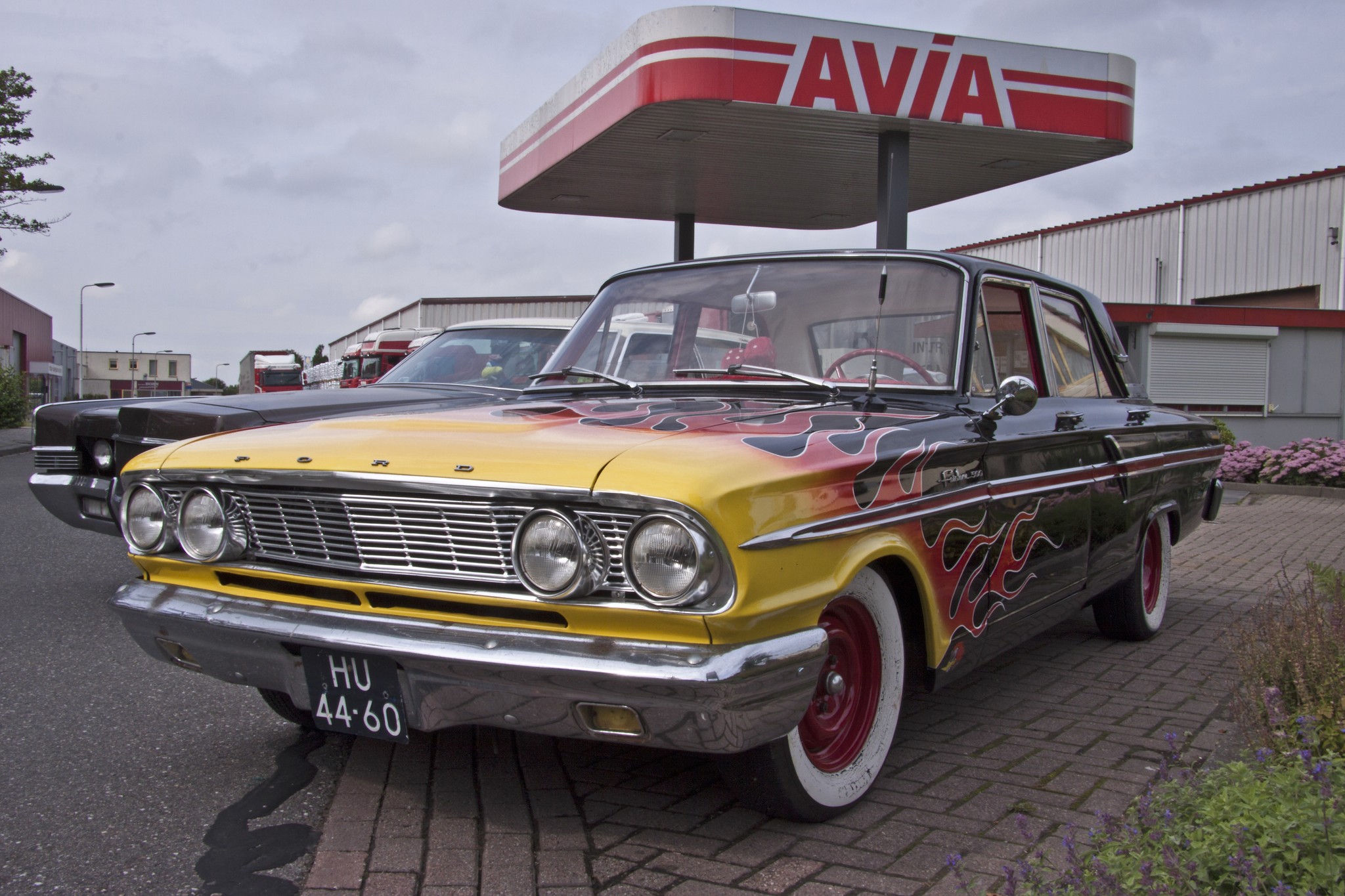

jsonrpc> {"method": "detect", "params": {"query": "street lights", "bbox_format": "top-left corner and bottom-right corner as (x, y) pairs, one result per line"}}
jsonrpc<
(154, 350), (173, 396)
(215, 363), (230, 396)
(131, 332), (156, 398)
(77, 282), (115, 399)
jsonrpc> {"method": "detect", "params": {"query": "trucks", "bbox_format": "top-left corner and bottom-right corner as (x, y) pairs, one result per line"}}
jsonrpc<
(238, 350), (307, 395)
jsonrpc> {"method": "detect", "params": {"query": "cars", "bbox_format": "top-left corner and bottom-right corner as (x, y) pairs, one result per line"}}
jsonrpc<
(27, 315), (761, 536)
(105, 247), (1233, 824)
